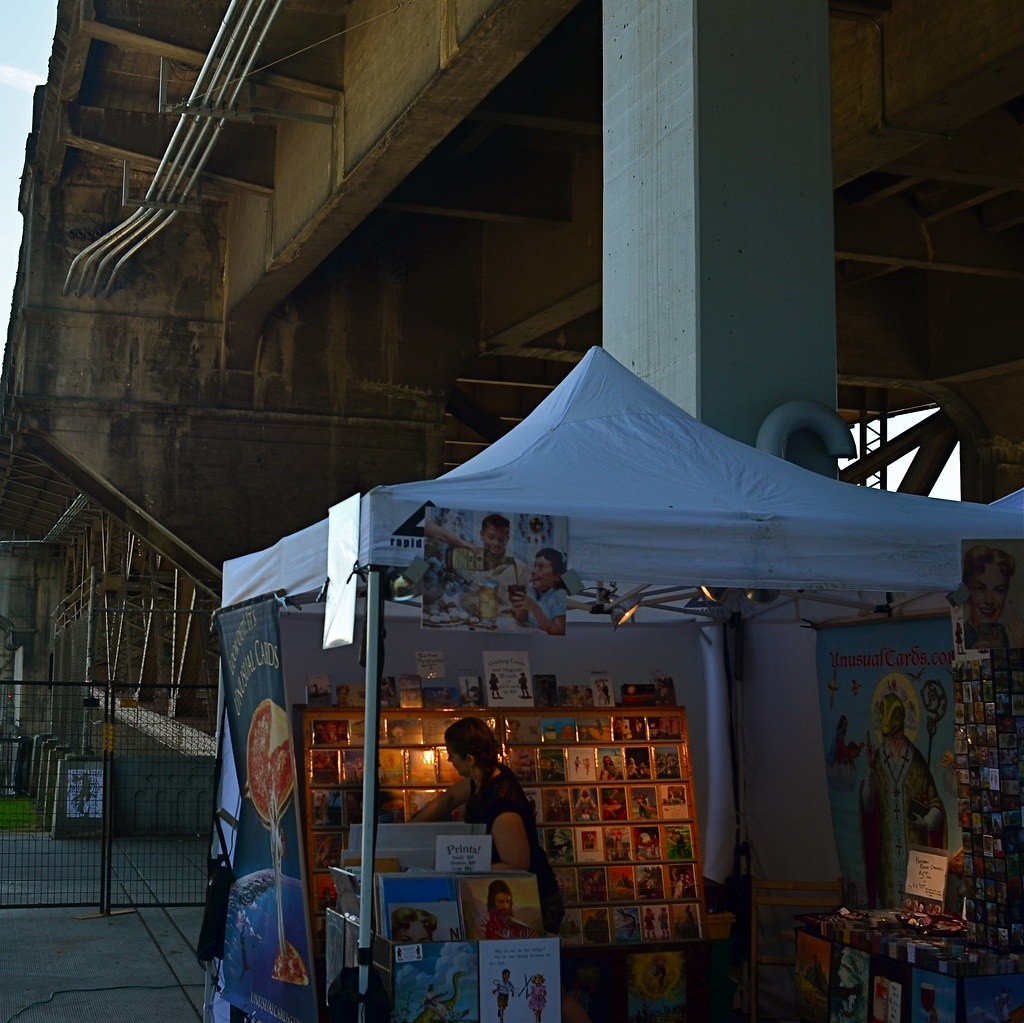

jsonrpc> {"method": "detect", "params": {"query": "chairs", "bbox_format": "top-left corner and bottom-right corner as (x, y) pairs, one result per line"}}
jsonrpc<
(751, 876), (844, 1023)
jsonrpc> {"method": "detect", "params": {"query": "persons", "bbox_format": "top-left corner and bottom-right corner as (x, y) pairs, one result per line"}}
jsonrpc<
(408, 718), (565, 937)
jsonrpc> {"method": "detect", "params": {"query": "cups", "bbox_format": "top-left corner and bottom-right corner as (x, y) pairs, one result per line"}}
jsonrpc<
(398, 674), (423, 709)
(509, 584), (526, 604)
(479, 580), (499, 626)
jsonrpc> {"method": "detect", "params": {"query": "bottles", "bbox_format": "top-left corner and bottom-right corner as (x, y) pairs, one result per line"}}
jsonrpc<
(445, 546), (515, 571)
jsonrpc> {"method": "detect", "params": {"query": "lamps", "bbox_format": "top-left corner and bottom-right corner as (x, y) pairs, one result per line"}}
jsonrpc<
(742, 588), (780, 605)
(701, 586), (729, 602)
(388, 568), (423, 602)
(600, 589), (644, 632)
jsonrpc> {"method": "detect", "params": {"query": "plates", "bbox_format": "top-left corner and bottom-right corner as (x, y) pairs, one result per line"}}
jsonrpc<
(423, 620), (469, 627)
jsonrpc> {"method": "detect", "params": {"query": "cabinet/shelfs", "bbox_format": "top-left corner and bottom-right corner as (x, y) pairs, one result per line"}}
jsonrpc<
(290, 700), (713, 1023)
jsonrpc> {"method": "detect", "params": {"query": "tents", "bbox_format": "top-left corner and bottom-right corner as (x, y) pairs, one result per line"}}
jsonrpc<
(203, 345), (1024, 1023)
